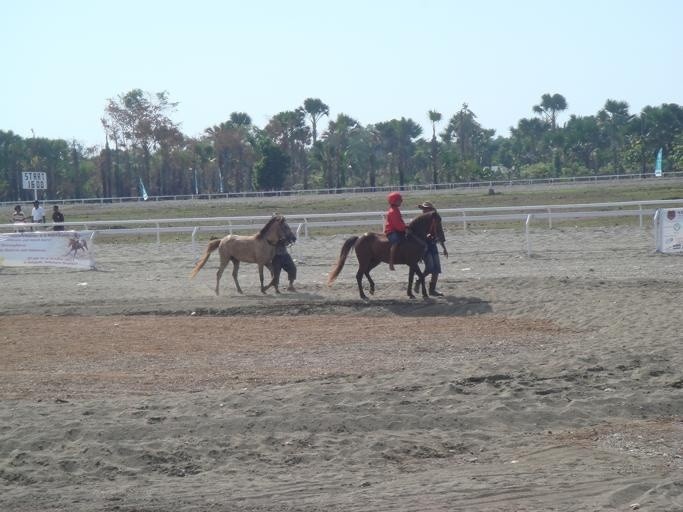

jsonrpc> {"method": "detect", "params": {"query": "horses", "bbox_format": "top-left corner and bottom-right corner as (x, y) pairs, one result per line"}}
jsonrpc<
(189, 211), (298, 295)
(325, 208), (446, 302)
(63, 238), (89, 261)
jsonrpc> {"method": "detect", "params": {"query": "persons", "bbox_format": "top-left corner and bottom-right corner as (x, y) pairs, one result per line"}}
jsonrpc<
(9, 205), (25, 231)
(272, 246), (296, 295)
(412, 201), (448, 295)
(384, 191), (407, 271)
(50, 205), (64, 230)
(25, 201), (45, 230)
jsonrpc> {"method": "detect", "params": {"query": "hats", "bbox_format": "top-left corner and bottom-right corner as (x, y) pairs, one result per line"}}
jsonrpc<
(418, 201), (434, 210)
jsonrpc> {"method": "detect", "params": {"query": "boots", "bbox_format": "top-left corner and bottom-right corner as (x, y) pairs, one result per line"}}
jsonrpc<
(414, 280), (421, 294)
(428, 281), (443, 296)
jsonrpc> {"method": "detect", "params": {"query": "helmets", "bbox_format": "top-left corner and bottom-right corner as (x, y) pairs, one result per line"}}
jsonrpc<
(387, 191), (401, 204)
